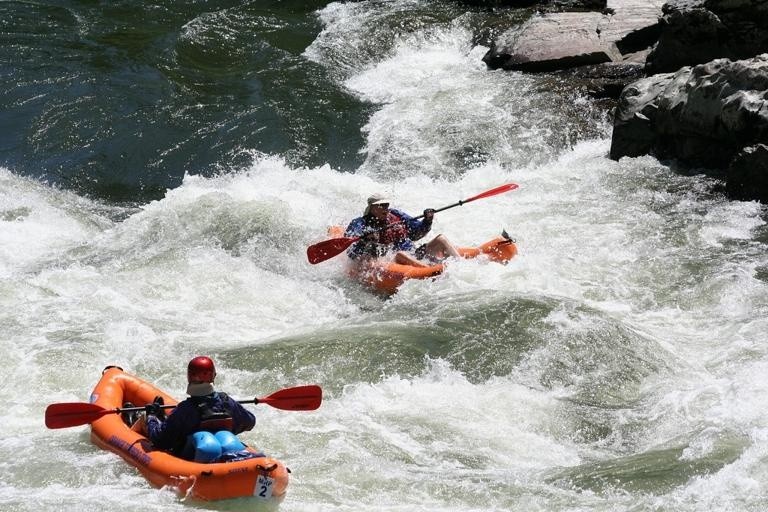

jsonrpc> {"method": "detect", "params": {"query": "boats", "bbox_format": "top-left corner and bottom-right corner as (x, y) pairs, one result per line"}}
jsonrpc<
(327, 225), (519, 298)
(88, 365), (293, 502)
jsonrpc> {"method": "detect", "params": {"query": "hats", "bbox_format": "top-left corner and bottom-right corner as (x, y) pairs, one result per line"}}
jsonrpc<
(367, 193), (391, 205)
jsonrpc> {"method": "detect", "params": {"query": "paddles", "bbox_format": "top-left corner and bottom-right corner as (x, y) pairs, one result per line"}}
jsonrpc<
(45, 385), (322, 429)
(307, 184), (518, 264)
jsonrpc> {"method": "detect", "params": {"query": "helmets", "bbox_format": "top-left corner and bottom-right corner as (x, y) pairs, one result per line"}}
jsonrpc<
(187, 356), (216, 383)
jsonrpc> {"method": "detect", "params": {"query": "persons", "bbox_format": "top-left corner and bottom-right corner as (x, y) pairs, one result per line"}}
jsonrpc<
(122, 353), (257, 462)
(340, 192), (465, 273)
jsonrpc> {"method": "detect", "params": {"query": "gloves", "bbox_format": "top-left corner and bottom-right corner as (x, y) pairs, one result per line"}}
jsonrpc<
(142, 401), (162, 418)
